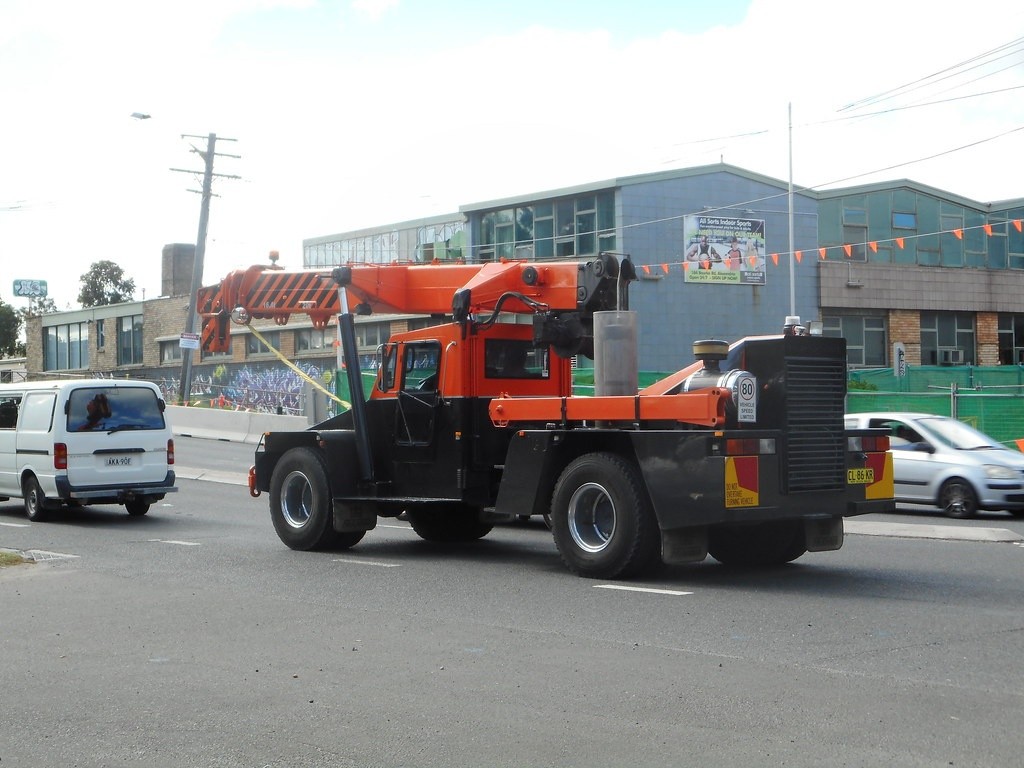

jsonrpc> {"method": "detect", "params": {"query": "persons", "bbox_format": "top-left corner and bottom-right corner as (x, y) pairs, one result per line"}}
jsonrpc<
(724, 237), (742, 270)
(743, 239), (758, 271)
(687, 235), (722, 270)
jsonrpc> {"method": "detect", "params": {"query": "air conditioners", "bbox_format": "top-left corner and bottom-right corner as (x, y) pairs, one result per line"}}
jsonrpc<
(941, 350), (964, 364)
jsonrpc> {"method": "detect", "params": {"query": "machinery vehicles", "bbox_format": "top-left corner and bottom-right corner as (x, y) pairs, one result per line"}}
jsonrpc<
(196, 250), (897, 580)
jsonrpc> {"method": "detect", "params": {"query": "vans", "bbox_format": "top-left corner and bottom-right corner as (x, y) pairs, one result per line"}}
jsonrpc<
(0, 380), (178, 522)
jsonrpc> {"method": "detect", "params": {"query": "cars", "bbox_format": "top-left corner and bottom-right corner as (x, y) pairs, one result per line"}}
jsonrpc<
(844, 412), (1024, 519)
